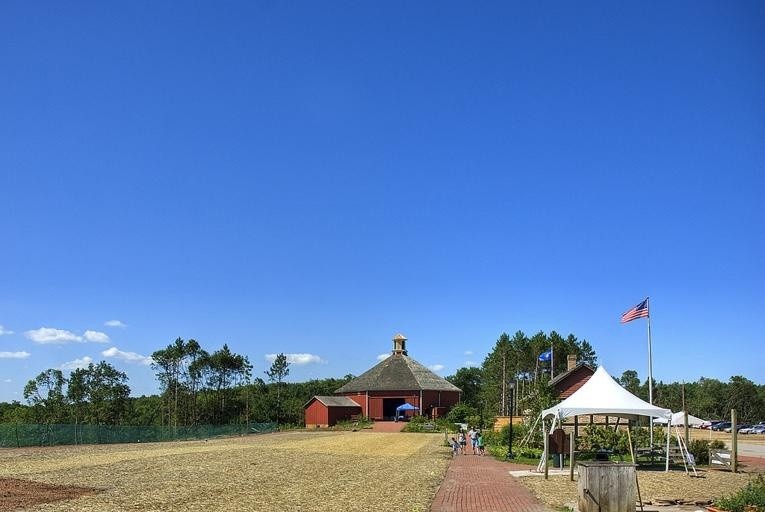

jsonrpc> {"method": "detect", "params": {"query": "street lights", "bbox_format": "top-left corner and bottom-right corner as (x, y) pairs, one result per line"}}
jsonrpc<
(480, 399), (484, 436)
(506, 381), (514, 460)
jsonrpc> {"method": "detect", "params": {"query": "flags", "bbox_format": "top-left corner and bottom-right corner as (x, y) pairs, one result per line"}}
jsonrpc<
(619, 296), (651, 325)
(537, 347), (552, 362)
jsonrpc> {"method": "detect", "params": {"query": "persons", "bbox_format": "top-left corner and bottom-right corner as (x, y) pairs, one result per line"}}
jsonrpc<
(468, 426), (481, 455)
(476, 433), (485, 457)
(456, 427), (467, 456)
(449, 437), (459, 456)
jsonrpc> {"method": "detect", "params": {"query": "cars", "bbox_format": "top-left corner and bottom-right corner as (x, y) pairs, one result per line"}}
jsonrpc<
(713, 423), (765, 434)
